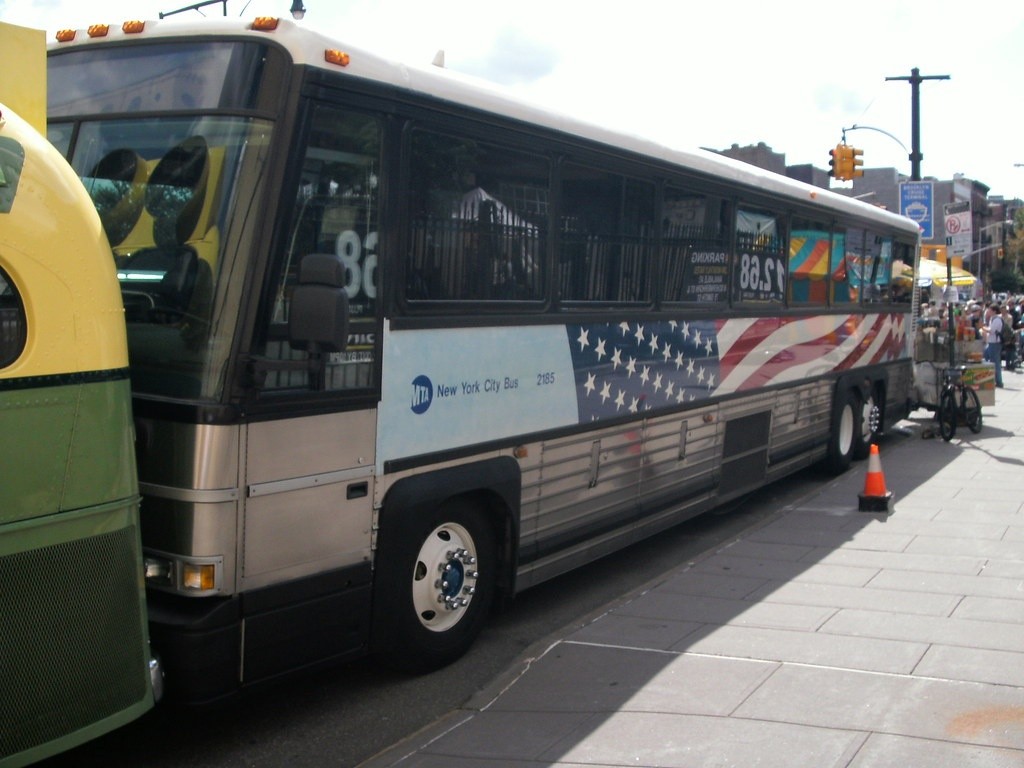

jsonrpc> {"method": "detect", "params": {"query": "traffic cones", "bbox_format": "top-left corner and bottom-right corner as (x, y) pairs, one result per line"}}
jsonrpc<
(858, 444), (892, 511)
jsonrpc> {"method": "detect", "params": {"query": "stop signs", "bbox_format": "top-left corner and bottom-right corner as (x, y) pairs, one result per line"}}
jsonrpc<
(943, 200), (972, 259)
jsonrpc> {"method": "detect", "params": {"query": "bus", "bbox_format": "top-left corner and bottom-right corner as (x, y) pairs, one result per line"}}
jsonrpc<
(46, 11), (923, 675)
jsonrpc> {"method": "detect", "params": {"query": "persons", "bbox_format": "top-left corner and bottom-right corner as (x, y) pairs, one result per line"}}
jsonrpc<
(982, 304), (1004, 388)
(919, 291), (1024, 371)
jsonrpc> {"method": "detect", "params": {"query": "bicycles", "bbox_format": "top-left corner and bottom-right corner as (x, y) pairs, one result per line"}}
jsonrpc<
(915, 358), (983, 442)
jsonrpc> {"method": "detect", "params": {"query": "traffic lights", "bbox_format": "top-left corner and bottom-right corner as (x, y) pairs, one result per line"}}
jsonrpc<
(844, 146), (865, 180)
(828, 144), (843, 181)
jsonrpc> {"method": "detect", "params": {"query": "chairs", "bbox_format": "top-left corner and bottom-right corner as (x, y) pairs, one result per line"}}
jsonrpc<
(270, 194), (370, 325)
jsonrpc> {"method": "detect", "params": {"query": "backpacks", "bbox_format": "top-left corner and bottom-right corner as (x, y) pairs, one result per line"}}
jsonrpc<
(991, 316), (1015, 347)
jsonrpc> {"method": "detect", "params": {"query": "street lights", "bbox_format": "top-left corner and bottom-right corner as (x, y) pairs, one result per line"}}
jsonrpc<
(979, 219), (1013, 281)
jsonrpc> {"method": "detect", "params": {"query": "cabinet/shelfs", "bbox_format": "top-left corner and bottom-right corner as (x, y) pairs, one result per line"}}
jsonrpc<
(954, 340), (984, 363)
(912, 360), (997, 408)
